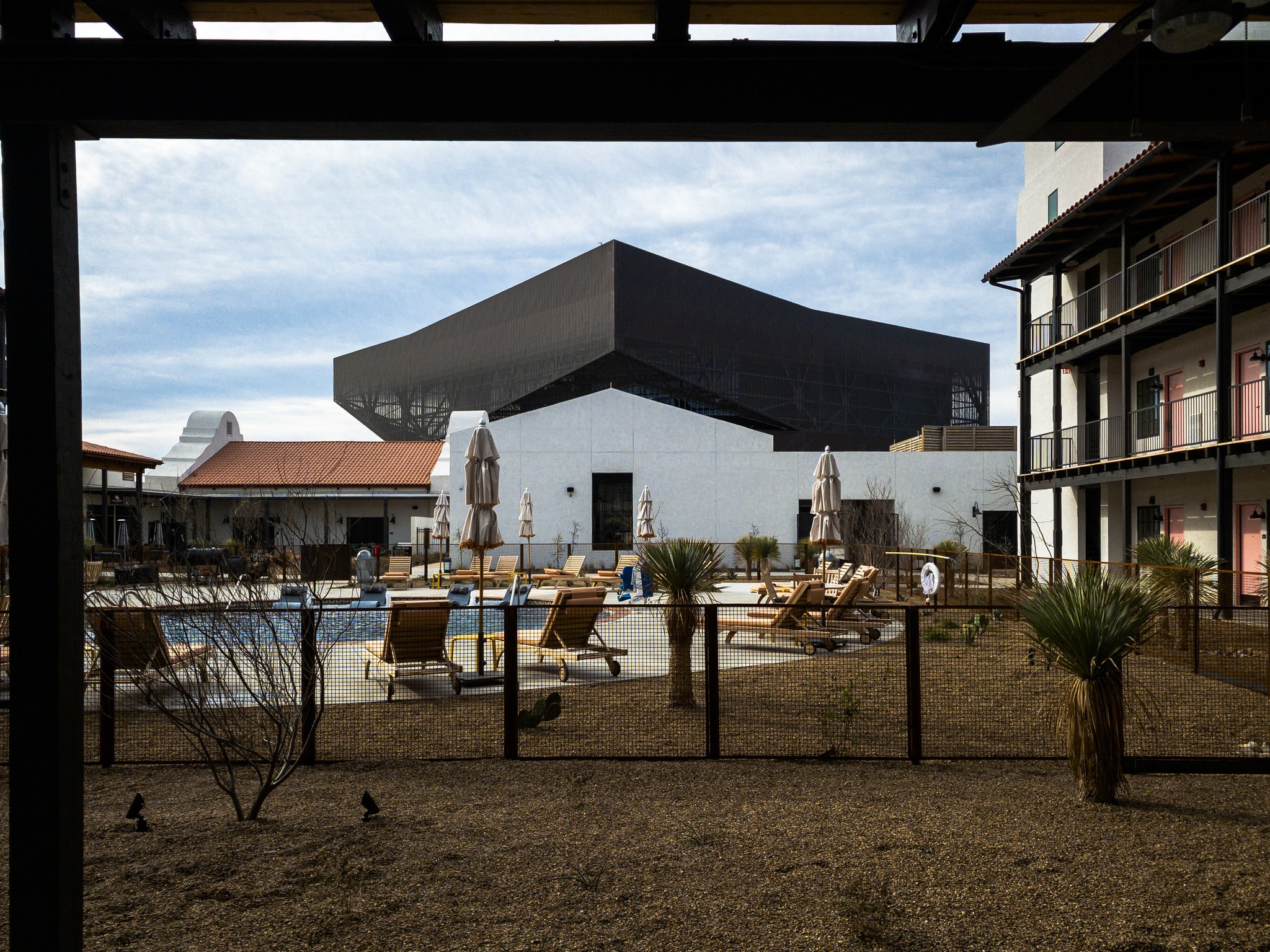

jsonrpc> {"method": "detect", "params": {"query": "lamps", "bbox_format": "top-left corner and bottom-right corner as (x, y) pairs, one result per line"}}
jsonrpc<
(1149, 376), (1165, 392)
(1150, 510), (1163, 522)
(1250, 505), (1266, 521)
(275, 514), (280, 525)
(1249, 346), (1266, 362)
(221, 513), (230, 525)
(972, 501), (982, 518)
(1143, 3), (1238, 53)
(336, 513), (344, 524)
(388, 513), (396, 524)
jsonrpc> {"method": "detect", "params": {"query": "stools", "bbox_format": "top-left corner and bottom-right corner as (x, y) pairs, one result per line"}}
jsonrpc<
(449, 634), (499, 672)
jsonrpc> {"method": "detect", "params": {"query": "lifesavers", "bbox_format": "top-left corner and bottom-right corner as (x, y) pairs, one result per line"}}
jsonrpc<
(920, 562), (941, 596)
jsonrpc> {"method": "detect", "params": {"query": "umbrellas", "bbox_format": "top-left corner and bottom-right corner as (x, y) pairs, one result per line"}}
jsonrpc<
(117, 521), (129, 567)
(86, 521), (96, 562)
(152, 522), (164, 564)
(432, 490), (450, 580)
(808, 445), (842, 632)
(458, 417), (505, 675)
(518, 487), (536, 585)
(636, 485), (657, 560)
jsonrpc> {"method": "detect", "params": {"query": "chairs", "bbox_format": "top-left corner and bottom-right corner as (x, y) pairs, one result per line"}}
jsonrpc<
(81, 603), (213, 705)
(82, 560), (103, 590)
(0, 594), (10, 683)
(363, 598), (464, 694)
(697, 559), (893, 653)
(380, 552), (640, 591)
(489, 584), (628, 681)
